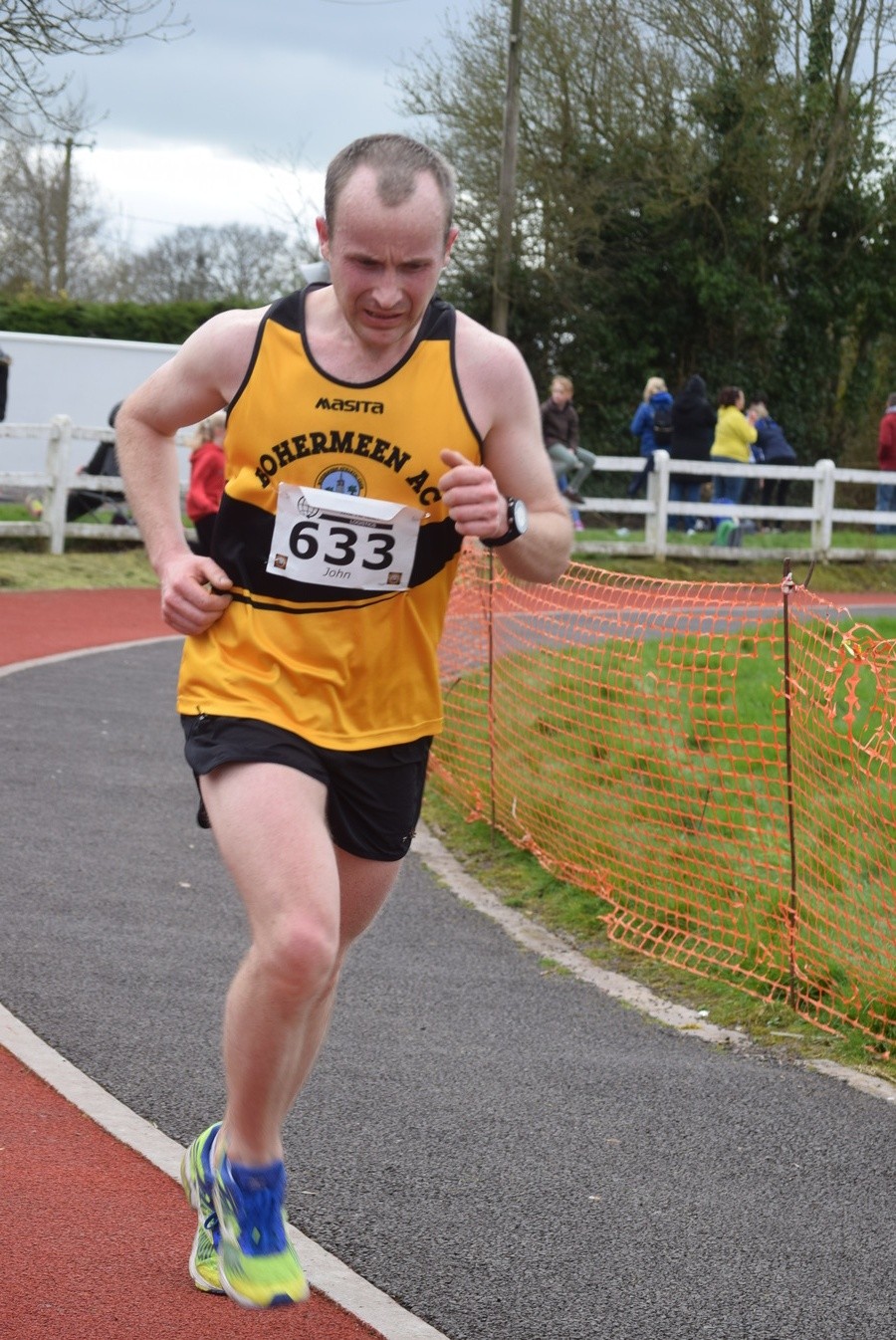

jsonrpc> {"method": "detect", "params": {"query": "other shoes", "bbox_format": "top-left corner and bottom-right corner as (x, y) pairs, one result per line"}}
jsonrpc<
(573, 519), (784, 538)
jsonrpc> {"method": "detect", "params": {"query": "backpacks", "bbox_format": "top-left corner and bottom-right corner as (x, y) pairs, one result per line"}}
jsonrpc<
(644, 402), (674, 447)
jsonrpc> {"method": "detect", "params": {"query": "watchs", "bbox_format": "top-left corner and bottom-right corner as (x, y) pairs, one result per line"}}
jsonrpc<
(479, 496), (528, 548)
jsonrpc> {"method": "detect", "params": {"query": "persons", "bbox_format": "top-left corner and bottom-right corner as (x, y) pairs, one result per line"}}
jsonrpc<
(874, 389), (896, 534)
(539, 375), (597, 504)
(112, 133), (577, 1312)
(26, 399), (122, 523)
(709, 386), (760, 527)
(666, 374), (719, 537)
(744, 401), (799, 533)
(629, 376), (677, 457)
(186, 410), (227, 557)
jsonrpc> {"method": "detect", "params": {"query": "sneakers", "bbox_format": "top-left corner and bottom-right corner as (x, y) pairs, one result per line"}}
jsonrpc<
(180, 1120), (289, 1295)
(208, 1124), (312, 1308)
